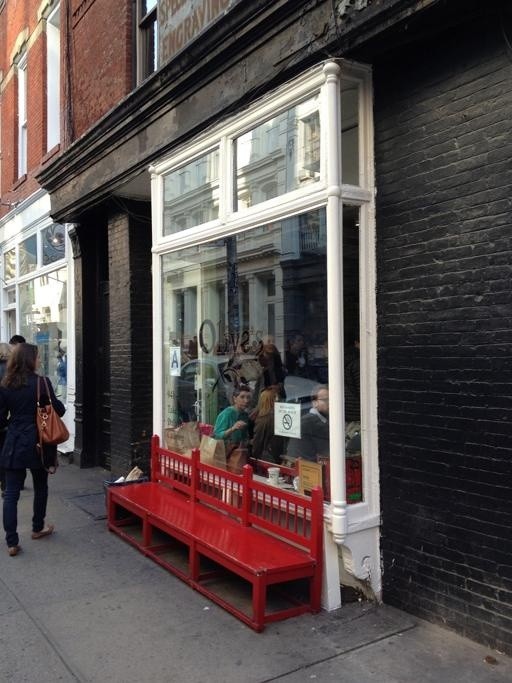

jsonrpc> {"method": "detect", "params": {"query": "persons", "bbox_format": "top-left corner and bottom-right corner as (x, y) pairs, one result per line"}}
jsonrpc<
(0, 335), (66, 557)
(188, 330), (361, 464)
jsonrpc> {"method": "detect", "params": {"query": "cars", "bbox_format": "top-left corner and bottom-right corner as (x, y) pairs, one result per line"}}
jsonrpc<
(174, 352), (327, 428)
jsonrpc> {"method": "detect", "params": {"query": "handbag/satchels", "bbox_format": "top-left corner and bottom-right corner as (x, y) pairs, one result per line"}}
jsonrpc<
(36, 406), (69, 445)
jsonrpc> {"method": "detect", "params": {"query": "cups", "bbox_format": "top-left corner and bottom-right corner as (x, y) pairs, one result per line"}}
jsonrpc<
(268, 467), (280, 485)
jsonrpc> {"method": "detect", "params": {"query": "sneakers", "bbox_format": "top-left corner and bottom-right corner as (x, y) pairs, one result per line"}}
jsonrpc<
(8, 546), (21, 555)
(31, 524), (53, 540)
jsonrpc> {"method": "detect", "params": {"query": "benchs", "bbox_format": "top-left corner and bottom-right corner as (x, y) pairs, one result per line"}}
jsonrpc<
(106, 433), (323, 633)
